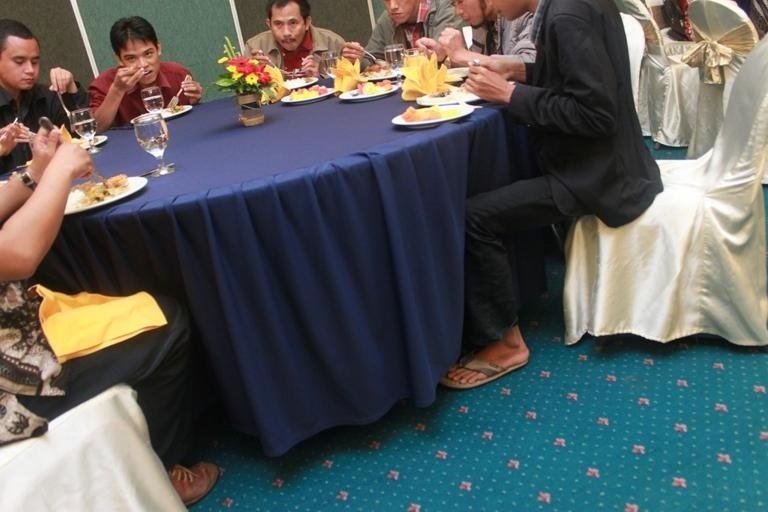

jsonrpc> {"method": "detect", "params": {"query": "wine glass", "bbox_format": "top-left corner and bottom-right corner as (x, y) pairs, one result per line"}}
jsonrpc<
(131, 111), (175, 178)
(382, 42), (406, 90)
(68, 107), (103, 156)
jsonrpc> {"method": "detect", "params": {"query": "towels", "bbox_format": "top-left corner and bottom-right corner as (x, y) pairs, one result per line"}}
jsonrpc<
(400, 50), (464, 102)
(27, 283), (167, 364)
(323, 55), (368, 96)
(261, 65), (289, 105)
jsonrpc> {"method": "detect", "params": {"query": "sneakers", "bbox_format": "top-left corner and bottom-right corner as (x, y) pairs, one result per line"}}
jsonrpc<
(167, 462), (219, 505)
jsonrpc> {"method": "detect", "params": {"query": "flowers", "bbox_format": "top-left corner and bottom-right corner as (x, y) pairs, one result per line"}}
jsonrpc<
(205, 35), (272, 94)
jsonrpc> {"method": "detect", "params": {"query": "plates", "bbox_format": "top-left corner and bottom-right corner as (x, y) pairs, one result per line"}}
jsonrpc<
(129, 103), (193, 125)
(280, 86), (337, 106)
(284, 75), (319, 89)
(416, 92), (482, 106)
(444, 65), (469, 79)
(390, 104), (474, 129)
(78, 134), (109, 151)
(360, 69), (397, 81)
(337, 85), (398, 103)
(63, 175), (147, 216)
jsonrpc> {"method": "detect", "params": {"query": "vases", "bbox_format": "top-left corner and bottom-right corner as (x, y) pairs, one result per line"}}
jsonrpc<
(237, 95), (265, 127)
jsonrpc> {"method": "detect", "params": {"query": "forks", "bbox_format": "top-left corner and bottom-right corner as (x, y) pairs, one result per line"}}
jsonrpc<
(166, 75), (191, 108)
(262, 53), (289, 75)
(56, 91), (75, 134)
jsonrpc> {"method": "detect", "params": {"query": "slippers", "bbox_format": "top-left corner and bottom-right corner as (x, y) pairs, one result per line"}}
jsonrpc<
(441, 349), (529, 390)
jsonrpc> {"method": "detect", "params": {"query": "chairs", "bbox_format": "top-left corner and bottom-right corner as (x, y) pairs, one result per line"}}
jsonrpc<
(0, 380), (190, 512)
(614, 0), (768, 185)
(565, 34), (767, 348)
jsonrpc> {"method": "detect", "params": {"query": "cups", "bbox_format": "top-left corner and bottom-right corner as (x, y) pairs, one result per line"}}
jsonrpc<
(407, 47), (426, 58)
(320, 50), (342, 78)
(140, 86), (165, 115)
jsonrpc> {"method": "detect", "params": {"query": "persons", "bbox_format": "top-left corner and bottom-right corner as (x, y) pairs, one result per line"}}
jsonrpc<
(86, 15), (203, 135)
(416, 0), (537, 68)
(0, 125), (220, 506)
(340, 0), (466, 73)
(241, 0), (346, 81)
(0, 17), (89, 179)
(437, 0), (665, 389)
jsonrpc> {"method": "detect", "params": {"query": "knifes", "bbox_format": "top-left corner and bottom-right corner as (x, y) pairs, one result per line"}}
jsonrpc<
(137, 162), (175, 178)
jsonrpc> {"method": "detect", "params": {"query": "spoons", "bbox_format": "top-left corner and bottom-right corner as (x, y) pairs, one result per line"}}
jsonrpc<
(360, 46), (385, 66)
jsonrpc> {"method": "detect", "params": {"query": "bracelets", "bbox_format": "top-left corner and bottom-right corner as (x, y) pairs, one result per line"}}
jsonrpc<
(18, 167), (38, 190)
